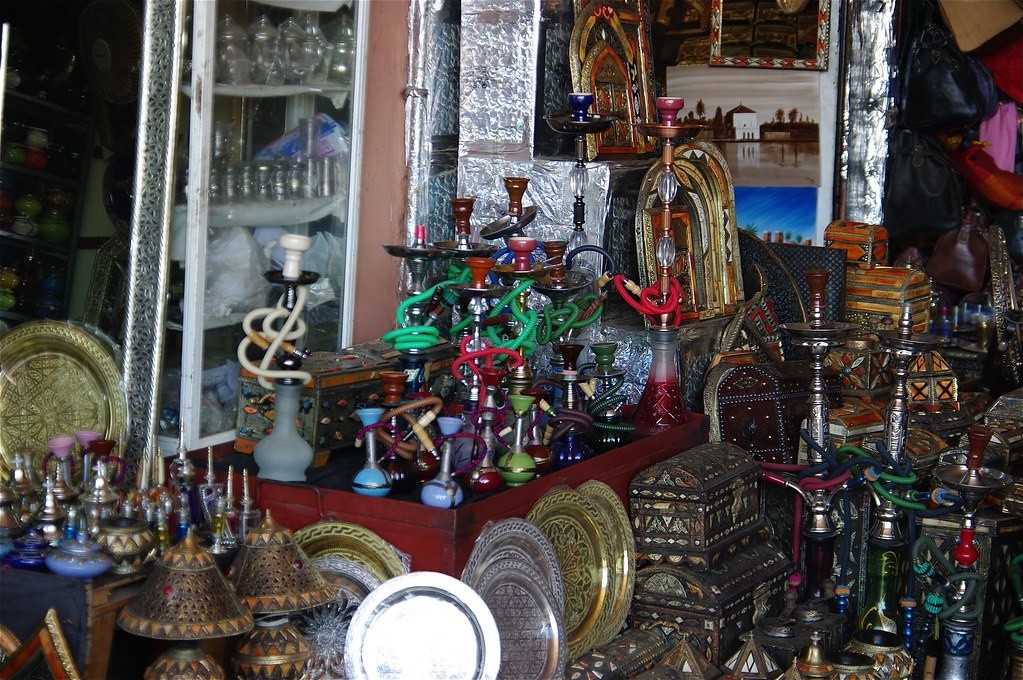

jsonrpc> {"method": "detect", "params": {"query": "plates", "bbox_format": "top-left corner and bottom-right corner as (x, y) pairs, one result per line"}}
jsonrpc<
(289, 520), (406, 680)
(461, 480), (636, 680)
(344, 570), (501, 680)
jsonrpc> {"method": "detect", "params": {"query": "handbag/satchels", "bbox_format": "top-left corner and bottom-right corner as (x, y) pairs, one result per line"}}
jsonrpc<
(903, 23), (986, 130)
(929, 205), (989, 292)
(884, 129), (966, 240)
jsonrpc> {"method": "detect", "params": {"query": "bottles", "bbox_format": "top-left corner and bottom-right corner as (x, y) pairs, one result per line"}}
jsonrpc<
(216, 10), (353, 86)
(253, 356), (314, 482)
(96, 518), (159, 573)
(631, 325), (693, 435)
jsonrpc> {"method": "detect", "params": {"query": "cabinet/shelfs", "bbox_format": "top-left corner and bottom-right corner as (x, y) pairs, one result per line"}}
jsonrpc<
(0, 85), (95, 327)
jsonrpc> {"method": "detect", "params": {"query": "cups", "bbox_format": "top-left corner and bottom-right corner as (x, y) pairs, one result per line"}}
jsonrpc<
(46, 530), (112, 581)
(207, 117), (333, 201)
